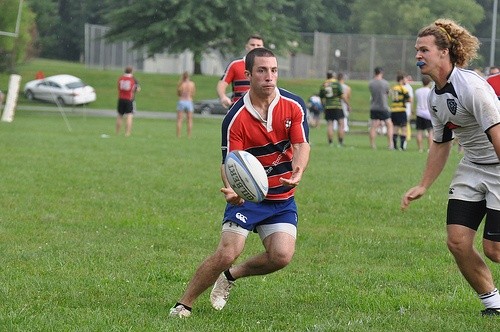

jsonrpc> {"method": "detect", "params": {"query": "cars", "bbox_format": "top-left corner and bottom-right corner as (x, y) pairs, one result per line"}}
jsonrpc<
(192, 92), (233, 116)
(22, 74), (97, 107)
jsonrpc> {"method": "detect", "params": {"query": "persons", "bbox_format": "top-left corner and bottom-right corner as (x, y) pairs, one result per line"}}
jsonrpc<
(216, 34), (269, 112)
(401, 18), (500, 317)
(306, 65), (500, 152)
(114, 64), (142, 136)
(168, 45), (310, 319)
(175, 69), (195, 138)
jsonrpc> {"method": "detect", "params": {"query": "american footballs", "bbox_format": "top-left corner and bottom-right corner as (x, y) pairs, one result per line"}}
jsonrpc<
(224, 150), (269, 203)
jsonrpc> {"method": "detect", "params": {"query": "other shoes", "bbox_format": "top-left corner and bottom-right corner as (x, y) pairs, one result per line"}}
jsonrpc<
(370, 145), (433, 153)
(329, 145), (332, 147)
(336, 144), (345, 148)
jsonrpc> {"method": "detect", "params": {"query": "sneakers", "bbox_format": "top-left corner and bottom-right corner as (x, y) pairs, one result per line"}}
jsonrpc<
(479, 307), (500, 318)
(208, 271), (238, 312)
(168, 303), (190, 320)
